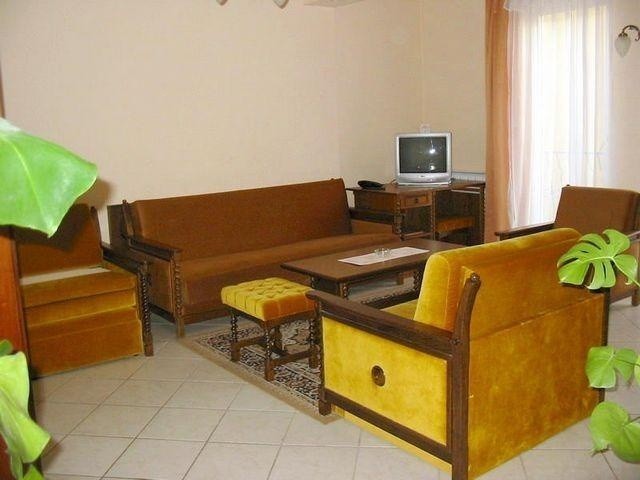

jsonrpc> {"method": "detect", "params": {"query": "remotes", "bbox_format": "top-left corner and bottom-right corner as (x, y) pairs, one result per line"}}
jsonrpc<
(358, 180), (382, 188)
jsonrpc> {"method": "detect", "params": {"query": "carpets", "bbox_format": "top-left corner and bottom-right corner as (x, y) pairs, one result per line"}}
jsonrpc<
(175, 282), (419, 426)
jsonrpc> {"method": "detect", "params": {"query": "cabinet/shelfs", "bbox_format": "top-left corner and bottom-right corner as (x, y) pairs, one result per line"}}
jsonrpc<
(342, 179), (486, 243)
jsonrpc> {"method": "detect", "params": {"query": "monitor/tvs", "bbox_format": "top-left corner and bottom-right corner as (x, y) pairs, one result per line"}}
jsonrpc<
(395, 132), (452, 186)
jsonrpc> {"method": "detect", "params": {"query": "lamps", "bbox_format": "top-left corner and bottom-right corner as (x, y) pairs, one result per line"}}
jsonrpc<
(615, 25), (640, 58)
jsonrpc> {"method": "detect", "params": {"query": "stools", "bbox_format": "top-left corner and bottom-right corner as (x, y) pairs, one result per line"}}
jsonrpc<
(219, 276), (318, 381)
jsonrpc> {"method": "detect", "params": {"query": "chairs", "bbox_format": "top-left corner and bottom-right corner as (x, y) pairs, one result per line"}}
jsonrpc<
(493, 185), (640, 310)
(304, 224), (611, 480)
(0, 203), (154, 380)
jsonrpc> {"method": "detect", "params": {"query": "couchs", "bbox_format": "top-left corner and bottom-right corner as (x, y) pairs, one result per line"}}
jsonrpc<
(104, 176), (405, 339)
